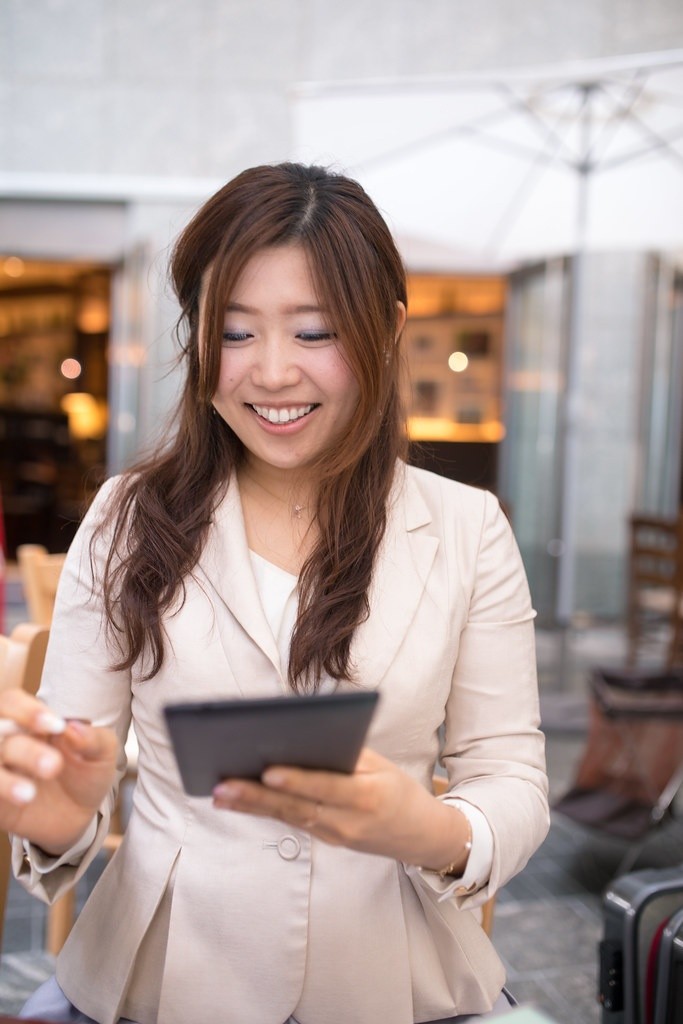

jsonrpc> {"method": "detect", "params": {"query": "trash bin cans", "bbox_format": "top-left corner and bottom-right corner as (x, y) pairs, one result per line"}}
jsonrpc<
(588, 670), (681, 795)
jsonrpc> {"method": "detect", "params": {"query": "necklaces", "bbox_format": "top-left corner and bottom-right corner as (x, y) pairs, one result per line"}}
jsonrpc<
(232, 469), (324, 518)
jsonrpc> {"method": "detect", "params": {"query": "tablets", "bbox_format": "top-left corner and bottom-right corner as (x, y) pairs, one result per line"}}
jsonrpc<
(163, 690), (381, 799)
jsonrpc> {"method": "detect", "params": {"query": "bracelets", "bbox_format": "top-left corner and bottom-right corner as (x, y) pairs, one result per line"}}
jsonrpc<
(413, 814), (472, 880)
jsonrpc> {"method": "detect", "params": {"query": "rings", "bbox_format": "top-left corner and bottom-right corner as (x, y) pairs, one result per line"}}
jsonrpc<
(298, 799), (324, 828)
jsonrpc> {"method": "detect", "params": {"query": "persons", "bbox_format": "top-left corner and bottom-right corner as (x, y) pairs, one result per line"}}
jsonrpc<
(0, 162), (551, 1023)
